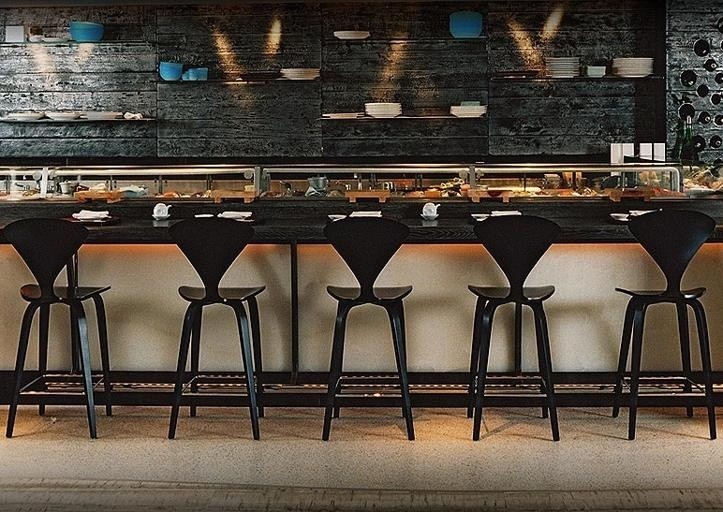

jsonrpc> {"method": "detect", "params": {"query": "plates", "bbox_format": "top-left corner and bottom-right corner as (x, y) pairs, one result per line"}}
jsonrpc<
(236, 219), (255, 222)
(611, 57), (652, 79)
(9, 111), (122, 122)
(280, 31), (490, 118)
(545, 57), (580, 79)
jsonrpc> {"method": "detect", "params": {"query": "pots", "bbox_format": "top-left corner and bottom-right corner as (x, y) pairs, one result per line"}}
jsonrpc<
(307, 176), (328, 188)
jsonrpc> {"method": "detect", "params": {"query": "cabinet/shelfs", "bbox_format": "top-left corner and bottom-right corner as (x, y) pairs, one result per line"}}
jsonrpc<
(0, 36), (665, 123)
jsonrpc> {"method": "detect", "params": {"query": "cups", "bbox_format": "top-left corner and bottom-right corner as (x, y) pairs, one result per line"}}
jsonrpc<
(182, 68), (208, 81)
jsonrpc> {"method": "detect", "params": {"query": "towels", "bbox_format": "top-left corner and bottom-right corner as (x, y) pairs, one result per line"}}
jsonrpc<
(491, 210), (521, 216)
(349, 211), (382, 217)
(217, 211), (253, 219)
(72, 210), (109, 220)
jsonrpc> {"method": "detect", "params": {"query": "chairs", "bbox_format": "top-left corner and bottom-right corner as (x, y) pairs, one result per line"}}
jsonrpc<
(322, 216), (415, 441)
(168, 217), (265, 440)
(4, 218), (111, 438)
(613, 210), (716, 440)
(468, 215), (561, 441)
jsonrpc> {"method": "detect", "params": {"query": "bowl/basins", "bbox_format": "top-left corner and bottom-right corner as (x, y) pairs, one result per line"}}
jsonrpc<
(152, 220), (169, 228)
(449, 11), (483, 37)
(159, 62), (183, 81)
(71, 21), (104, 41)
(587, 66), (606, 78)
(195, 214), (213, 218)
(328, 214), (346, 221)
(420, 214), (439, 220)
(421, 220), (438, 227)
(152, 214), (170, 221)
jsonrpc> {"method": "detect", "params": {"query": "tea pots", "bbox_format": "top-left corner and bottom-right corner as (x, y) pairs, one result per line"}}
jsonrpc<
(153, 203), (172, 216)
(422, 202), (441, 215)
